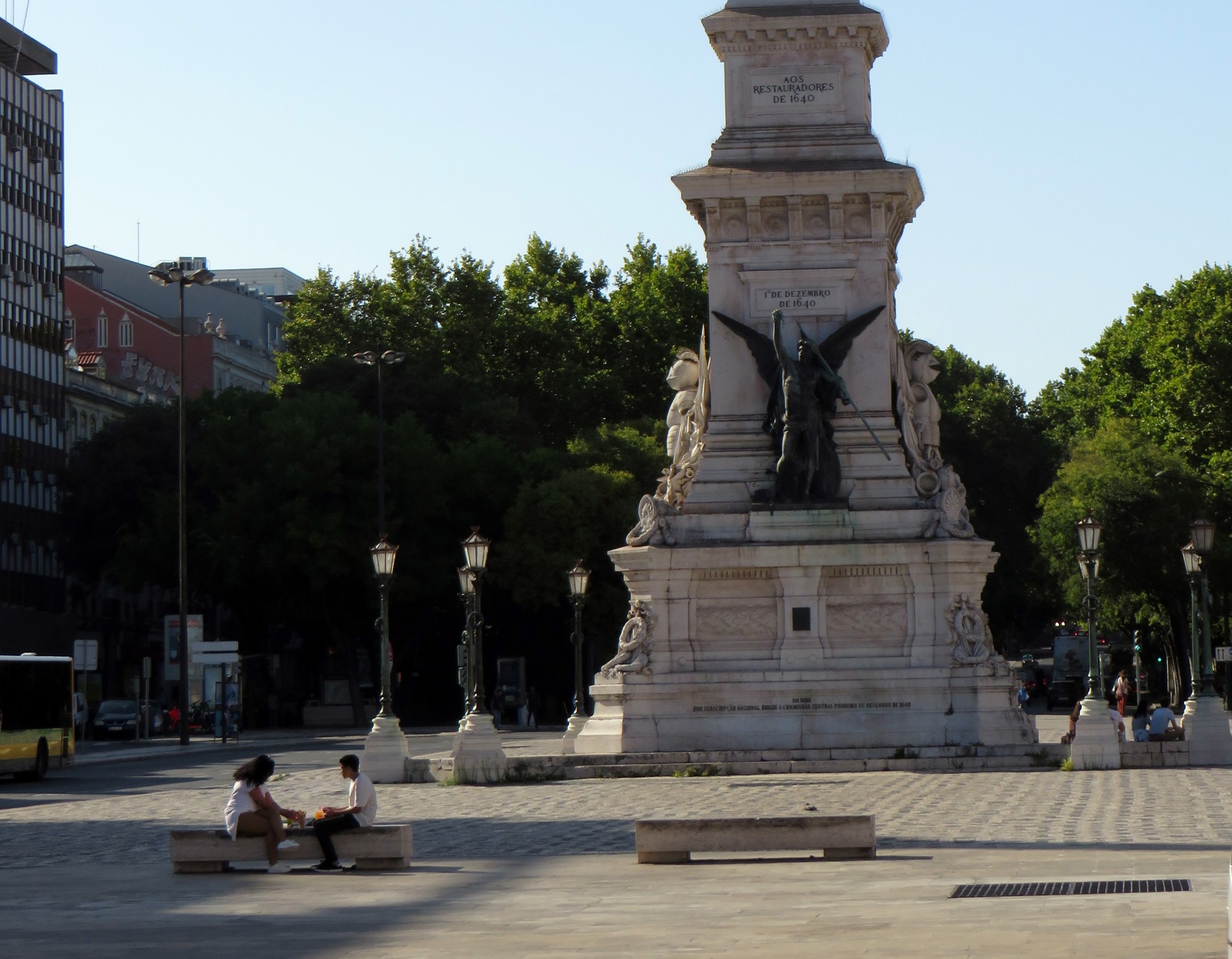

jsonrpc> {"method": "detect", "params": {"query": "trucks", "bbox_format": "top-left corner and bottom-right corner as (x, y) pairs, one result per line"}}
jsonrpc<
(1050, 635), (1092, 686)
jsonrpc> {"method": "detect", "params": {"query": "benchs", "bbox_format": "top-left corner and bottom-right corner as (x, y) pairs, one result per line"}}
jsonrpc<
(170, 824), (412, 875)
(635, 814), (877, 865)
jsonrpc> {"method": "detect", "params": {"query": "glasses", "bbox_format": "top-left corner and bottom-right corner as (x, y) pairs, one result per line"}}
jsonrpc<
(266, 756), (272, 762)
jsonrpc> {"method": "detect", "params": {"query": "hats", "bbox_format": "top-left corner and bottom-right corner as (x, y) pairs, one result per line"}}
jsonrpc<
(1119, 670), (1128, 676)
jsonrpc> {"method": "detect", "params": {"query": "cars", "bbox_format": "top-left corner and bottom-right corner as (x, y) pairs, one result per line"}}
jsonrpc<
(1110, 649), (1149, 697)
(1013, 655), (1048, 695)
(73, 693), (90, 730)
(1074, 631), (1105, 646)
(94, 699), (173, 740)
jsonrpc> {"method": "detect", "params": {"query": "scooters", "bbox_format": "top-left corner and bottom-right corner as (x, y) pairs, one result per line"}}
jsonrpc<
(168, 700), (243, 736)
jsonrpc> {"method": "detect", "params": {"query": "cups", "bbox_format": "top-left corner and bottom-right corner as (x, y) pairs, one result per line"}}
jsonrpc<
(315, 811), (324, 819)
(287, 818), (294, 827)
(298, 814), (306, 829)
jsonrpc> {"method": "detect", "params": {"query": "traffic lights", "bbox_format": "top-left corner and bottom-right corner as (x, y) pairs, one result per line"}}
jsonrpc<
(1133, 630), (1143, 653)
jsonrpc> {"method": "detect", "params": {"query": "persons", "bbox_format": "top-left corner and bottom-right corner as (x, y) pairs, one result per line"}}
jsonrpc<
(904, 340), (941, 461)
(1016, 678), (1029, 712)
(1109, 670), (1132, 715)
(306, 754), (378, 871)
(1061, 699), (1084, 744)
(169, 706), (180, 731)
(1132, 698), (1151, 742)
(491, 689), (501, 723)
(1108, 708), (1125, 742)
(526, 686), (541, 728)
(226, 753), (299, 873)
(1111, 649), (1133, 668)
(772, 309), (850, 505)
(665, 347), (699, 458)
(1149, 696), (1185, 742)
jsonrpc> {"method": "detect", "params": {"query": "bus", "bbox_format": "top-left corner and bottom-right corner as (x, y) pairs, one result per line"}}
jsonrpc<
(0, 653), (79, 781)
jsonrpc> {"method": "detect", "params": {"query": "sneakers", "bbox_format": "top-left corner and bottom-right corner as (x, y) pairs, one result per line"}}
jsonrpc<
(277, 839), (299, 851)
(268, 863), (291, 873)
(311, 859), (342, 872)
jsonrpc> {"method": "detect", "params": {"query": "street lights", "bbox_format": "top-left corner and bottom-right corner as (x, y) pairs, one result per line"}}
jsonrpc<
(146, 265), (215, 747)
(1180, 540), (1203, 700)
(456, 564), (477, 716)
(462, 524), (491, 714)
(565, 559), (592, 718)
(1074, 506), (1107, 700)
(1187, 512), (1222, 696)
(368, 538), (398, 717)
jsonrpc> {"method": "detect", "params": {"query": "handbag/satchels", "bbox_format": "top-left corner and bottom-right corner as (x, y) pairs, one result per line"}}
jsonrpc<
(1115, 694), (1120, 701)
(1116, 679), (1123, 697)
(1112, 685), (1118, 695)
